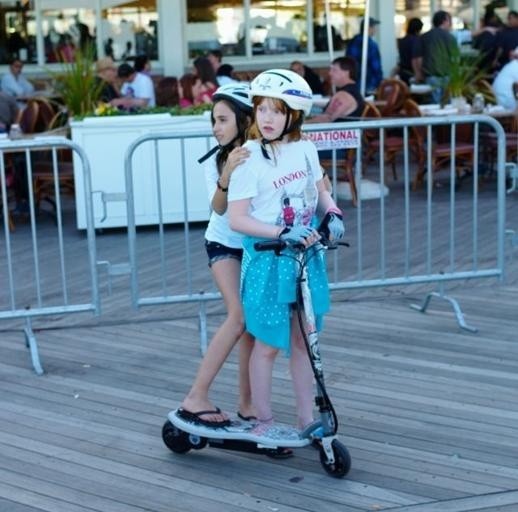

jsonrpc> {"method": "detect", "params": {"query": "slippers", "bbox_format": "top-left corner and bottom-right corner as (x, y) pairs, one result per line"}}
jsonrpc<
(177, 405), (232, 428)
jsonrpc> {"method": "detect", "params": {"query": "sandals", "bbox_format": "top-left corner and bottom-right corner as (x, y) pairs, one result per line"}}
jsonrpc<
(266, 448), (293, 459)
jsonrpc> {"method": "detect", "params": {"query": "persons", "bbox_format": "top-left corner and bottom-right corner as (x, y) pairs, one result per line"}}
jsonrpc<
(227, 67), (345, 460)
(1, 5), (236, 161)
(313, 5), (518, 115)
(303, 58), (366, 160)
(488, 37), (518, 193)
(177, 81), (258, 429)
(291, 59), (328, 97)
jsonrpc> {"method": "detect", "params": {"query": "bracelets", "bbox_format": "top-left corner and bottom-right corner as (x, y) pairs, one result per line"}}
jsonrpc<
(217, 178), (227, 192)
(326, 207), (342, 215)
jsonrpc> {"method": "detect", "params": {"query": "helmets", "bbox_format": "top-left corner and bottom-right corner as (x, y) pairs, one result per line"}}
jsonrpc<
(212, 82), (253, 113)
(250, 68), (314, 117)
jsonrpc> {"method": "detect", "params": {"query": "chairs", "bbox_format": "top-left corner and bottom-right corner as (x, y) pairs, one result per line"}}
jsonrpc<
(317, 70), (518, 206)
(2, 90), (76, 228)
